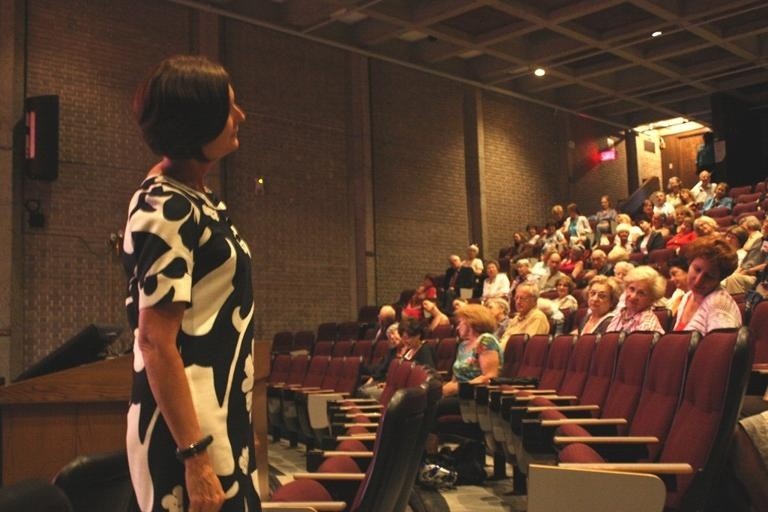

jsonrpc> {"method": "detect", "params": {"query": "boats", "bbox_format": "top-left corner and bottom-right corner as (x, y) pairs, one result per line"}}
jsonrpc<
(24, 94), (59, 180)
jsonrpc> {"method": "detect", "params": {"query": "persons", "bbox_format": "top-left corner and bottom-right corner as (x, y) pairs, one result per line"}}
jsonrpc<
(396, 171), (764, 510)
(118, 52), (268, 510)
(360, 306), (436, 420)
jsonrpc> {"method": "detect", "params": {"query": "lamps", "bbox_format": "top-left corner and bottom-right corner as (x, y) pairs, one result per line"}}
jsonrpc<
(0, 476), (73, 512)
(53, 448), (140, 512)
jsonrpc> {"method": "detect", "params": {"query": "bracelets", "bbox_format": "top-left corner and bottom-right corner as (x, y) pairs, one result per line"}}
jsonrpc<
(174, 435), (214, 461)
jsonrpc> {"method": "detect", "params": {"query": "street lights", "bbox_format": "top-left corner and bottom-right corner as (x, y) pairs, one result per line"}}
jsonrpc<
(489, 377), (538, 386)
(417, 433), (488, 490)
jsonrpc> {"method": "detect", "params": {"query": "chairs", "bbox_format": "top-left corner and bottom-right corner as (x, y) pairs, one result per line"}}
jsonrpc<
(0, 476), (73, 512)
(558, 327), (757, 508)
(431, 299), (706, 478)
(262, 287), (443, 510)
(53, 448), (140, 512)
(627, 182), (768, 397)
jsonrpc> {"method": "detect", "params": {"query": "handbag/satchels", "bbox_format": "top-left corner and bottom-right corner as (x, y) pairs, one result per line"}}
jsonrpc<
(174, 435), (214, 461)
